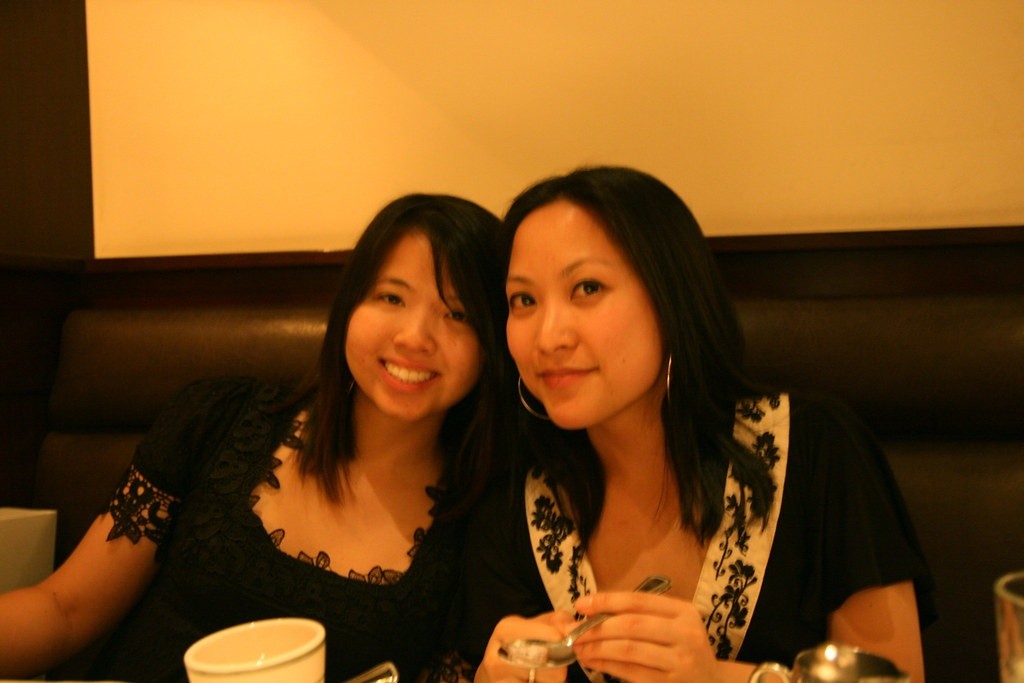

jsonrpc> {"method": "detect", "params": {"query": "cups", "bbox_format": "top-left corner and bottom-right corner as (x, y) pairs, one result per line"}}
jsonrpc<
(992, 571), (1024, 683)
(182, 616), (326, 683)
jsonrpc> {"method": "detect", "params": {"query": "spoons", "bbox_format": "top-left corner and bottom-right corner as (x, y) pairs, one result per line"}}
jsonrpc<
(497, 573), (673, 668)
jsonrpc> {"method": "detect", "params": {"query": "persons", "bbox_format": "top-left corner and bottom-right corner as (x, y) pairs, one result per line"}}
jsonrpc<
(463, 167), (941, 683)
(0, 192), (500, 683)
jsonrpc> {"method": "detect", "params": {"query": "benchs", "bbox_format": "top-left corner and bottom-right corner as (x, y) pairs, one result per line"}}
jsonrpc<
(29, 299), (1023, 683)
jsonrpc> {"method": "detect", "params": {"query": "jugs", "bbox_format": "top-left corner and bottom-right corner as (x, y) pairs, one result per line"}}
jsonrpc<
(748, 641), (909, 683)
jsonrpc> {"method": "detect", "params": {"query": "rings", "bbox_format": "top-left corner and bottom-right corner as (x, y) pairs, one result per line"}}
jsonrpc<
(528, 668), (536, 683)
